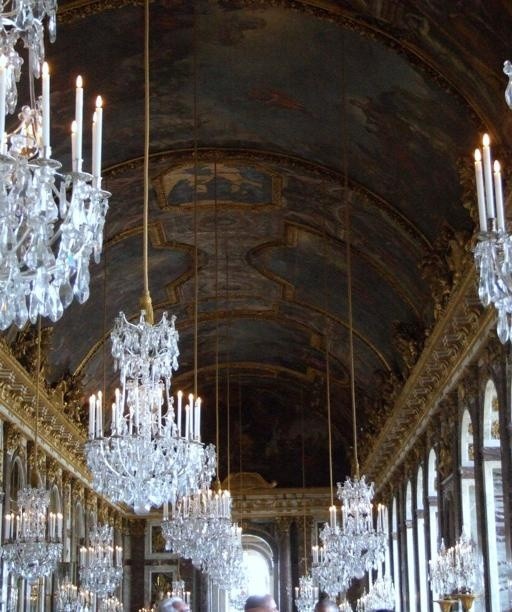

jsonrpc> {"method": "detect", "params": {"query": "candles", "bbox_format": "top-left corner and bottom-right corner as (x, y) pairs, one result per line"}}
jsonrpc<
(471, 131), (505, 233)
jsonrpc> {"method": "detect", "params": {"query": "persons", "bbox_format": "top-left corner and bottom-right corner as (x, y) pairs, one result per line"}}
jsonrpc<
(313, 600), (339, 612)
(159, 596), (190, 612)
(244, 595), (275, 612)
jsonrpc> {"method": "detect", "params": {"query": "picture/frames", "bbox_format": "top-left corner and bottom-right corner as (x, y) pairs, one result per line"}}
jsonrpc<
(142, 562), (182, 610)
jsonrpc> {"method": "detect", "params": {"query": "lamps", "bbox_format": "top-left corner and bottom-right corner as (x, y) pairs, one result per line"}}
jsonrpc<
(72, 361), (125, 596)
(157, 68), (231, 596)
(309, 19), (394, 600)
(2, 345), (66, 590)
(1, 2), (122, 342)
(427, 534), (482, 612)
(83, 5), (219, 515)
